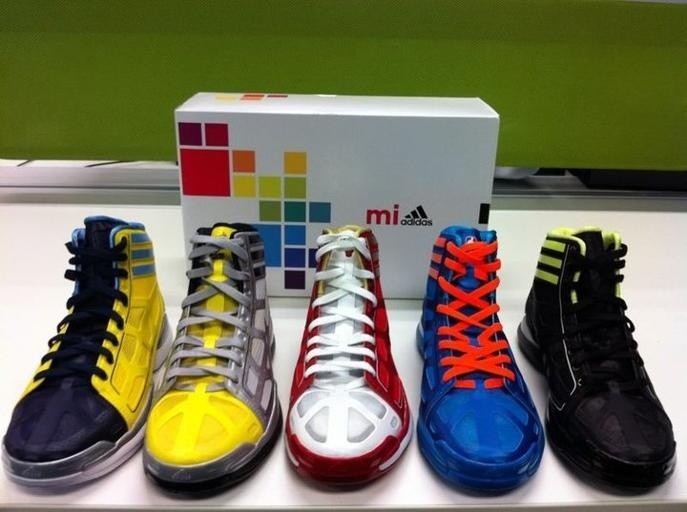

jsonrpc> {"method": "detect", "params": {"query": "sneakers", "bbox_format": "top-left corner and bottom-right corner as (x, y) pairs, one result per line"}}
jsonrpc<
(416, 225), (544, 496)
(1, 216), (173, 487)
(142, 223), (281, 494)
(284, 225), (412, 490)
(518, 227), (677, 495)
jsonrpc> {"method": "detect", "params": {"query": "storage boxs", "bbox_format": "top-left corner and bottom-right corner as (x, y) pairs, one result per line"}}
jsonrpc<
(170, 89), (503, 306)
